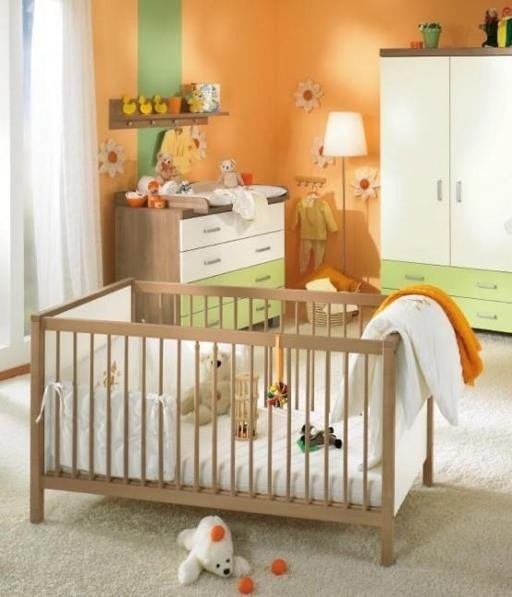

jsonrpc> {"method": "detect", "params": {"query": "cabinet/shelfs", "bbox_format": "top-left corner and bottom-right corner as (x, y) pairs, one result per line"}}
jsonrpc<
(115, 190), (288, 331)
(379, 47), (511, 333)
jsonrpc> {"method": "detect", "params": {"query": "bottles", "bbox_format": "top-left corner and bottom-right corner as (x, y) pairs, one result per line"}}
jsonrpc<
(147, 182), (166, 209)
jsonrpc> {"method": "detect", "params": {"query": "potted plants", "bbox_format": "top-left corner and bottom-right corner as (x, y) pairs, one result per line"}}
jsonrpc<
(418, 18), (442, 48)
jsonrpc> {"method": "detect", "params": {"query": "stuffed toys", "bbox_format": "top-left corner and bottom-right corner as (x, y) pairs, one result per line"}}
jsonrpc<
(180, 347), (242, 427)
(479, 6), (512, 49)
(215, 159), (245, 190)
(155, 152), (176, 187)
(297, 423), (343, 454)
(175, 514), (252, 586)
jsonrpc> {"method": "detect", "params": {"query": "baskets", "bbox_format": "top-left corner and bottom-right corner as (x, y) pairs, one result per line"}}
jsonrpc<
(305, 286), (353, 328)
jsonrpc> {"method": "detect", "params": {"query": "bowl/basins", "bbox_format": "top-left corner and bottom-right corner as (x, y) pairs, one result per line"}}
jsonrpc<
(125, 196), (147, 207)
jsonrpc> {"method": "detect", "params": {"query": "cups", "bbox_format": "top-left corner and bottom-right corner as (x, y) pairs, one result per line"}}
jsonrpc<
(168, 97), (182, 115)
(241, 172), (253, 185)
(422, 29), (441, 49)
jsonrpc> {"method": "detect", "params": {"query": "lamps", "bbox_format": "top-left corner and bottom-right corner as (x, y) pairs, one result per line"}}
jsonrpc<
(322, 109), (370, 272)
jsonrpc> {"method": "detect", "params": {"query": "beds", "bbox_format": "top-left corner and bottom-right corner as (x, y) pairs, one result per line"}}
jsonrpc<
(31, 276), (433, 567)
